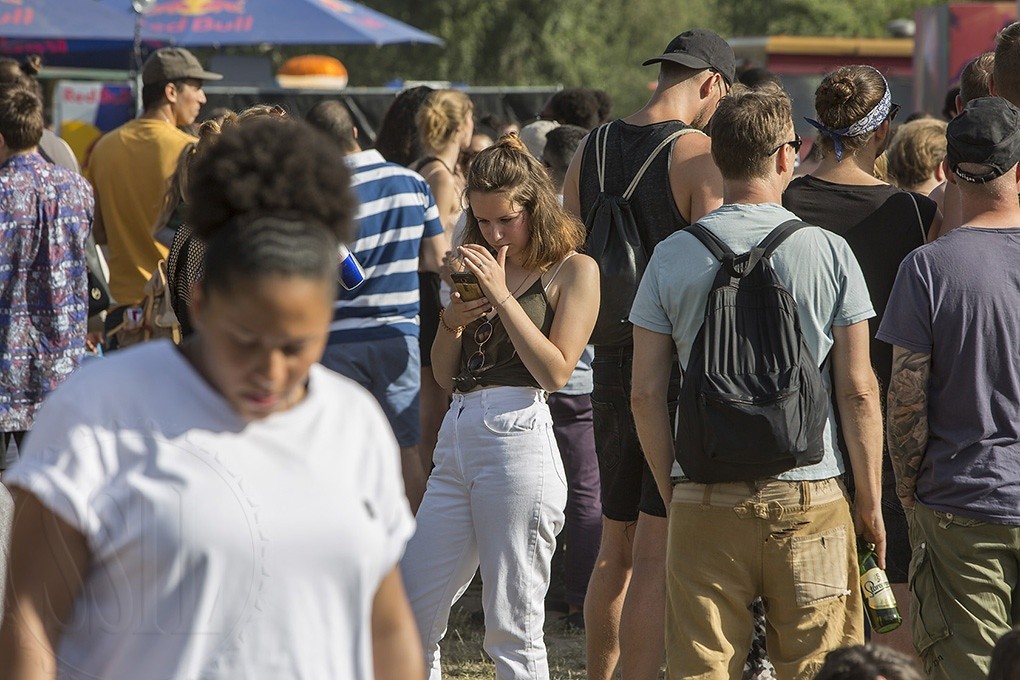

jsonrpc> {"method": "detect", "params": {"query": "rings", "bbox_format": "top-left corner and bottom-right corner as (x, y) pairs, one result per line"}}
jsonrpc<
(476, 261), (483, 267)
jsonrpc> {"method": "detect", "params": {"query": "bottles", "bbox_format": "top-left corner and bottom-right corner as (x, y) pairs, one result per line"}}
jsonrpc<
(856, 535), (902, 633)
(335, 239), (367, 292)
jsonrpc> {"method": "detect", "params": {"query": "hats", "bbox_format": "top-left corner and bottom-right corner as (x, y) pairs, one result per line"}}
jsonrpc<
(945, 96), (1020, 184)
(643, 29), (736, 87)
(142, 48), (224, 85)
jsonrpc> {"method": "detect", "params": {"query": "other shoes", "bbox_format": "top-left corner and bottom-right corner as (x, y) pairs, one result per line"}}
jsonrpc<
(566, 612), (584, 628)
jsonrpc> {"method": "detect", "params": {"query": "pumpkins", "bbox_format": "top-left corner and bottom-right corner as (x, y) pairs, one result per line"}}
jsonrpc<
(279, 53), (347, 76)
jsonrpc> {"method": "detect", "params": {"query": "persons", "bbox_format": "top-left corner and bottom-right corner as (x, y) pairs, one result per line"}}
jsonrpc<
(0, 115), (425, 679)
(876, 95), (1020, 680)
(627, 80), (886, 679)
(399, 129), (602, 679)
(0, 22), (1020, 680)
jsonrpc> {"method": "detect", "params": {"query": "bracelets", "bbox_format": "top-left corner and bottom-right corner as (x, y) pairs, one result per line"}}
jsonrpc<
(438, 308), (467, 334)
(494, 292), (512, 308)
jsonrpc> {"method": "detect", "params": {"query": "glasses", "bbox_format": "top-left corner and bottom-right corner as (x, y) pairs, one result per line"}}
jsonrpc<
(466, 318), (493, 373)
(768, 132), (802, 156)
(873, 102), (901, 132)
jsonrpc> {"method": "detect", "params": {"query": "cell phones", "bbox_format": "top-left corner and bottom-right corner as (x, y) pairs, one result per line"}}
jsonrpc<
(450, 272), (492, 313)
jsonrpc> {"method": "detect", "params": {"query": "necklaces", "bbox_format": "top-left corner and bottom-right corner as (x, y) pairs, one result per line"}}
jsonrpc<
(485, 269), (533, 314)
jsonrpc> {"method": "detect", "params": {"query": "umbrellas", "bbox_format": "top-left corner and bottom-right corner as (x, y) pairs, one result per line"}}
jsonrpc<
(0, 0), (448, 52)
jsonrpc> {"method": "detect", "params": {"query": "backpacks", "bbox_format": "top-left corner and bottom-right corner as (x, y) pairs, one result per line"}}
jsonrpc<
(674, 219), (830, 484)
(577, 121), (706, 348)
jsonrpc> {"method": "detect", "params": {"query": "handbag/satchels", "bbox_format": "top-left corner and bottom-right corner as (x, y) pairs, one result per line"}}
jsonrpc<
(106, 256), (182, 348)
(84, 258), (110, 319)
